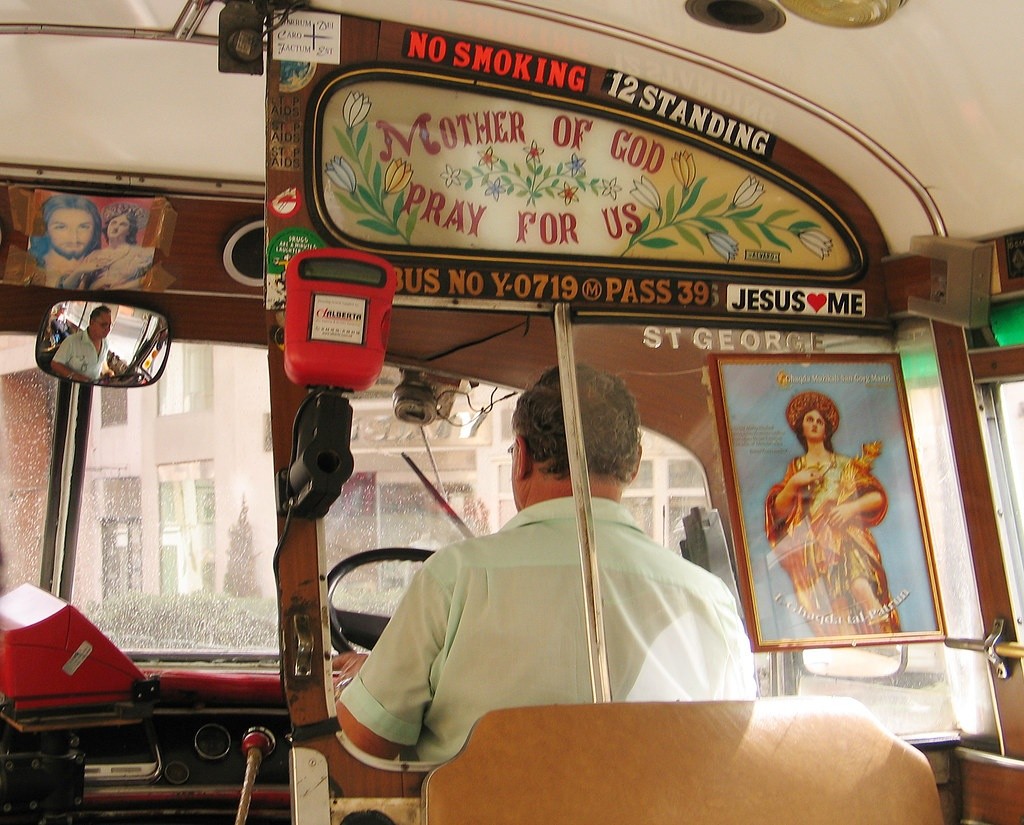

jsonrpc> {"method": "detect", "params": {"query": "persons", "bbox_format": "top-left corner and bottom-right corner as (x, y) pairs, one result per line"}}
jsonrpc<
(51, 303), (71, 344)
(333, 361), (755, 764)
(51, 306), (115, 383)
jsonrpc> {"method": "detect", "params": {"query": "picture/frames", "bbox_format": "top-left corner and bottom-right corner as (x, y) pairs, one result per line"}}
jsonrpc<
(705, 350), (949, 652)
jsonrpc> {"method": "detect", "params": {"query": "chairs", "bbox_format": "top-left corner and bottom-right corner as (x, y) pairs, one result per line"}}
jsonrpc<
(418, 697), (946, 825)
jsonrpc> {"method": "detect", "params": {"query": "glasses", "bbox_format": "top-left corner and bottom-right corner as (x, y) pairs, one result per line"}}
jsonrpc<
(94, 321), (110, 328)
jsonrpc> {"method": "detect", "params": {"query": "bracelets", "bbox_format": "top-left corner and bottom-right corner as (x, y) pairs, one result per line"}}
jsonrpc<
(66, 372), (74, 380)
(334, 677), (353, 696)
